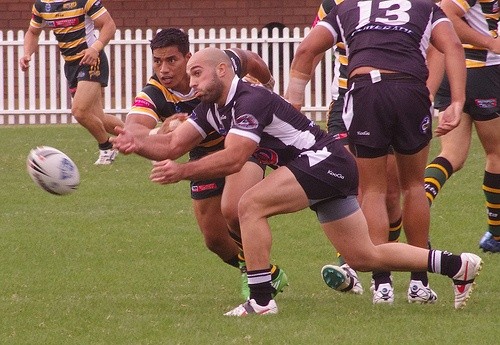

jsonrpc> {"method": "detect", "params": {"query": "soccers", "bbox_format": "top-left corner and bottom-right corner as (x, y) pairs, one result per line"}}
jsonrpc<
(25, 146), (81, 195)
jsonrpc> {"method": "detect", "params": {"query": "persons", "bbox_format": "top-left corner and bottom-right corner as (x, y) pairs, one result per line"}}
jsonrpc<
(20, 0), (125, 165)
(125, 29), (290, 302)
(109, 48), (484, 318)
(285, 0), (500, 304)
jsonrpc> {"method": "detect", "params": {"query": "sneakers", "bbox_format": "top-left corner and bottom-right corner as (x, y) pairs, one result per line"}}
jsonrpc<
(249, 264), (289, 300)
(93, 147), (118, 167)
(452, 252), (484, 311)
(480, 231), (500, 253)
(406, 279), (438, 305)
(235, 254), (252, 298)
(370, 278), (394, 306)
(223, 298), (280, 318)
(319, 262), (364, 297)
